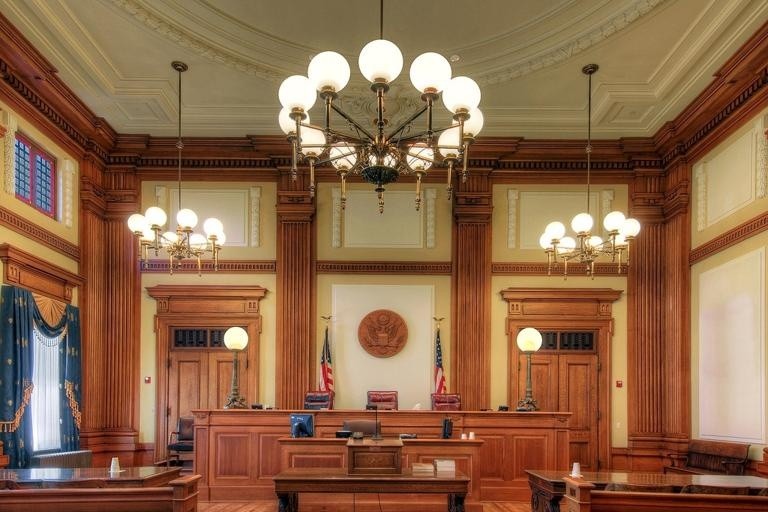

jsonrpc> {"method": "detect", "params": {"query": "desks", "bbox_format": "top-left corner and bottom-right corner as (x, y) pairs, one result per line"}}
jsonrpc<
(276, 435), (485, 512)
(1, 466), (184, 491)
(520, 469), (768, 511)
(272, 467), (471, 512)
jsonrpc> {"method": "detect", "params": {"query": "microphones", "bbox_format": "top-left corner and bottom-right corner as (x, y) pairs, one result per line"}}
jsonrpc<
(375, 405), (378, 435)
(444, 419), (449, 438)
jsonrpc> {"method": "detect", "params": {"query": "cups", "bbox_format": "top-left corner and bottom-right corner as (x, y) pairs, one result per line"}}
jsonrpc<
(110, 457), (120, 472)
(571, 462), (581, 478)
(461, 432), (476, 440)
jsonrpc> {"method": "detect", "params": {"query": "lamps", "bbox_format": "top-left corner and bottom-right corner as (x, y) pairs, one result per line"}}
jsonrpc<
(273, 3), (487, 219)
(222, 326), (250, 408)
(127, 61), (228, 281)
(533, 68), (642, 282)
(515, 326), (543, 411)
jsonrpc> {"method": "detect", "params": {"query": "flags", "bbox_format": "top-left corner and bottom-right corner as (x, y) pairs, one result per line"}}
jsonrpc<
(432, 321), (446, 395)
(319, 329), (336, 412)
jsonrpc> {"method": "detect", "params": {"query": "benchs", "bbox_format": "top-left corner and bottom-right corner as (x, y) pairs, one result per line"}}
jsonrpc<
(662, 438), (752, 475)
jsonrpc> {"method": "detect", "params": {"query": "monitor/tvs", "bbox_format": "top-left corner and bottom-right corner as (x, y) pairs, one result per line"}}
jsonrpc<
(290, 413), (314, 436)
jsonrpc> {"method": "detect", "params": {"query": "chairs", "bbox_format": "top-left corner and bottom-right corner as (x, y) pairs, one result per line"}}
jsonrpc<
(303, 390), (463, 410)
(343, 418), (381, 437)
(166, 416), (194, 474)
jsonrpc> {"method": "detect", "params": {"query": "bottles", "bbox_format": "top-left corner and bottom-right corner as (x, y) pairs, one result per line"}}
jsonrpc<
(446, 418), (453, 438)
